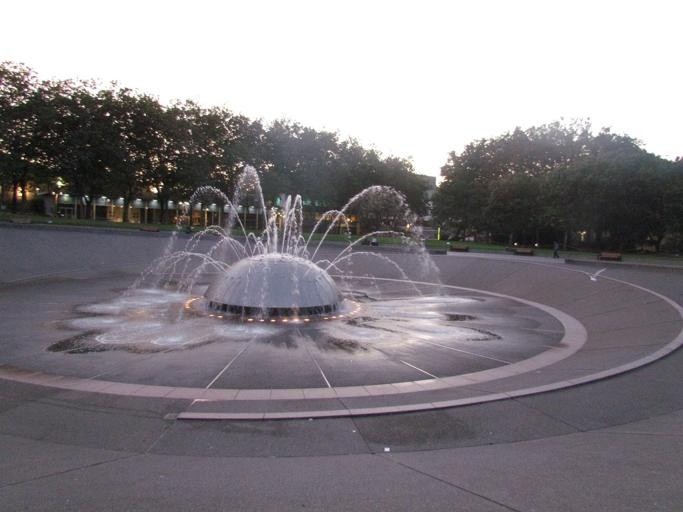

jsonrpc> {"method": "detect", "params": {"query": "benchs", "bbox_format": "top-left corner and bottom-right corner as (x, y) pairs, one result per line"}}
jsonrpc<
(597, 251), (621, 261)
(513, 247), (532, 256)
(450, 244), (468, 252)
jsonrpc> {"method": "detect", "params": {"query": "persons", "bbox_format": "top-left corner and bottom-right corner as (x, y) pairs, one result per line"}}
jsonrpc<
(553, 240), (560, 259)
